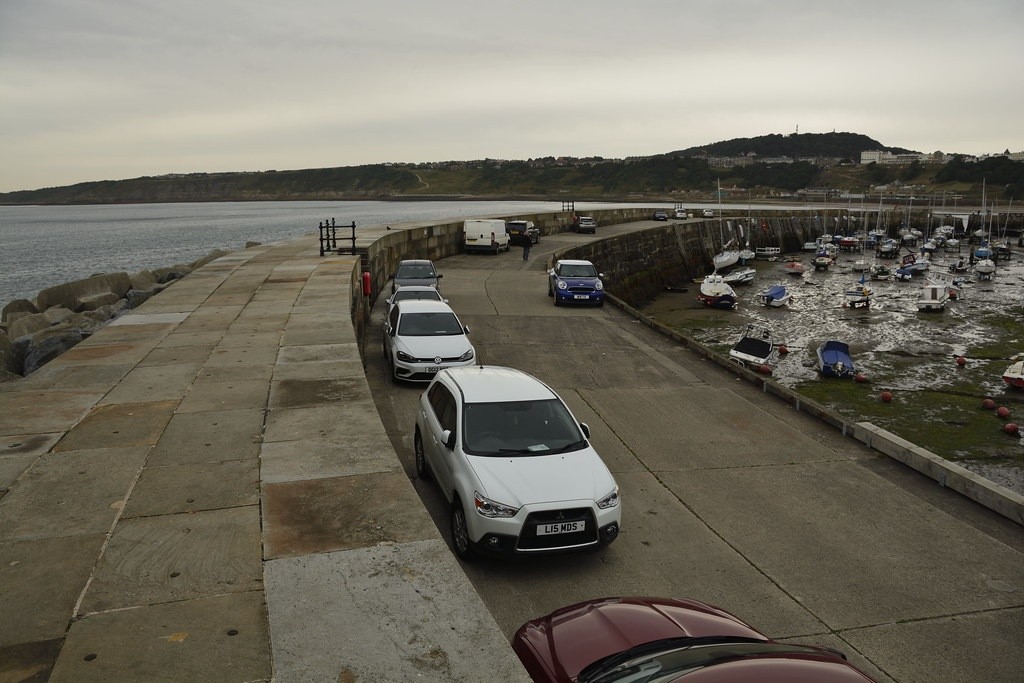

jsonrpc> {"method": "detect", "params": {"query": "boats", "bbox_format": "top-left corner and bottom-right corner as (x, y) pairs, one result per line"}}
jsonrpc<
(817, 340), (855, 379)
(840, 282), (875, 310)
(757, 282), (792, 307)
(697, 173), (1024, 300)
(728, 322), (775, 371)
(914, 284), (951, 312)
(1001, 360), (1024, 389)
(699, 272), (738, 307)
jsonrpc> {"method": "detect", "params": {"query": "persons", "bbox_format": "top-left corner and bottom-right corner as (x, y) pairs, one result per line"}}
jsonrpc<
(520, 232), (533, 261)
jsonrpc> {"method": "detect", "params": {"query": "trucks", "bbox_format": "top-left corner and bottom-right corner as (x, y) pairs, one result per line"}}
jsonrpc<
(463, 219), (511, 255)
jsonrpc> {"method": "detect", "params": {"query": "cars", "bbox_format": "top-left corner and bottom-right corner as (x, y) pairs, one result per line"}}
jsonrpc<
(653, 210), (669, 221)
(700, 208), (714, 218)
(510, 594), (878, 683)
(385, 285), (450, 313)
(380, 299), (477, 384)
(388, 260), (444, 294)
(546, 259), (607, 308)
(672, 209), (687, 220)
(413, 364), (623, 563)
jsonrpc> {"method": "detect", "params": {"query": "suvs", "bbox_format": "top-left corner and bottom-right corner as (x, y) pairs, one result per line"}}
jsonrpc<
(506, 219), (540, 247)
(567, 216), (596, 234)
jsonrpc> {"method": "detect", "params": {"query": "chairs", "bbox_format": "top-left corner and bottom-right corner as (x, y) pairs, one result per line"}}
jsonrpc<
(517, 409), (546, 439)
